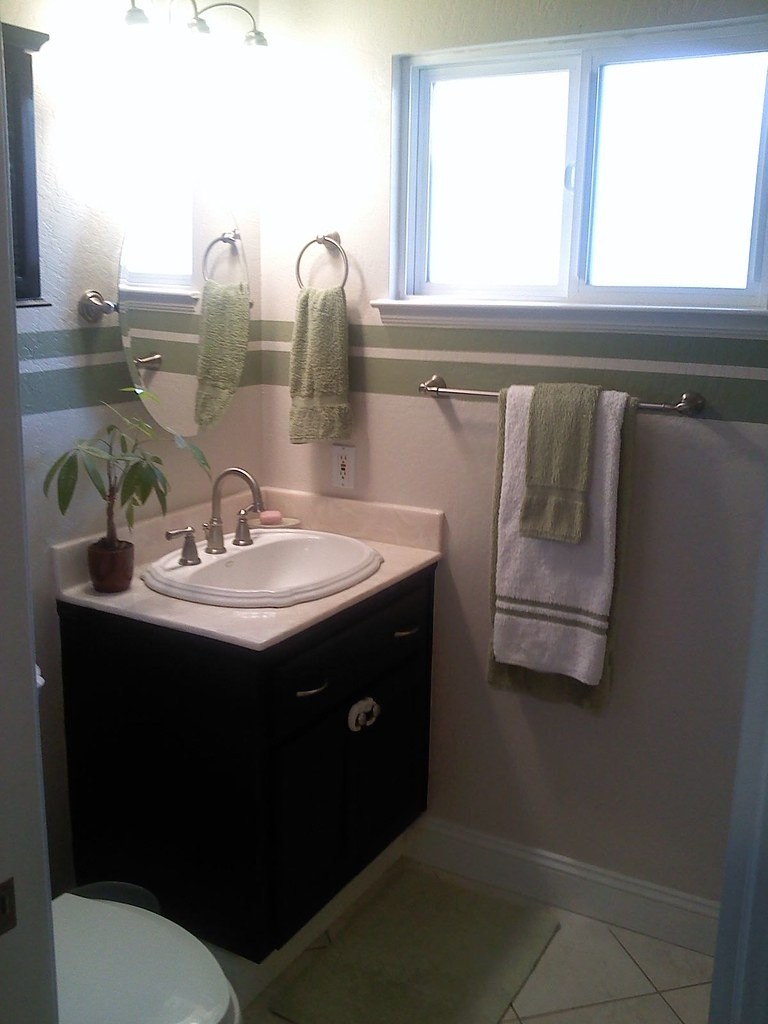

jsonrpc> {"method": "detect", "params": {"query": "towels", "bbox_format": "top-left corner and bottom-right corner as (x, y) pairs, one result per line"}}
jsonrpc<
(287, 286), (353, 446)
(194, 279), (253, 428)
(486, 381), (638, 713)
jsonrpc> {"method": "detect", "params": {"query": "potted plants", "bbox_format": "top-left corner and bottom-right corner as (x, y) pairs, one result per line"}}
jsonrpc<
(47, 390), (213, 593)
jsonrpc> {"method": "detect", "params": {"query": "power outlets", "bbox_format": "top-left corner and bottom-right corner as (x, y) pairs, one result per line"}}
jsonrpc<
(329, 444), (354, 489)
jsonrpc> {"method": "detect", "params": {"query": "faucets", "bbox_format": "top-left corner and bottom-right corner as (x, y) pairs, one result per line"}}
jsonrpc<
(202, 466), (265, 554)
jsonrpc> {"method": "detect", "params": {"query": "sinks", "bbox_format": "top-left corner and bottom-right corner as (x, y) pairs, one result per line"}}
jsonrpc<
(139, 528), (385, 608)
(260, 510), (282, 525)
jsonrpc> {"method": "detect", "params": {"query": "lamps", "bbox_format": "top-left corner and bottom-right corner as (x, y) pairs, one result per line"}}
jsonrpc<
(127, 0), (268, 51)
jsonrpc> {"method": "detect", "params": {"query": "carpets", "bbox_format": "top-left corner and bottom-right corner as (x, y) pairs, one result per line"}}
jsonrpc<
(270, 867), (560, 1024)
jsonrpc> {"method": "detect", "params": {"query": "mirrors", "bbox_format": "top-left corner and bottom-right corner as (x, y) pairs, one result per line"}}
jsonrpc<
(118, 189), (251, 437)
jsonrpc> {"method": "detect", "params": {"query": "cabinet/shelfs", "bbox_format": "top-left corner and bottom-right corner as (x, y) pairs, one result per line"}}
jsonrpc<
(55, 560), (439, 965)
